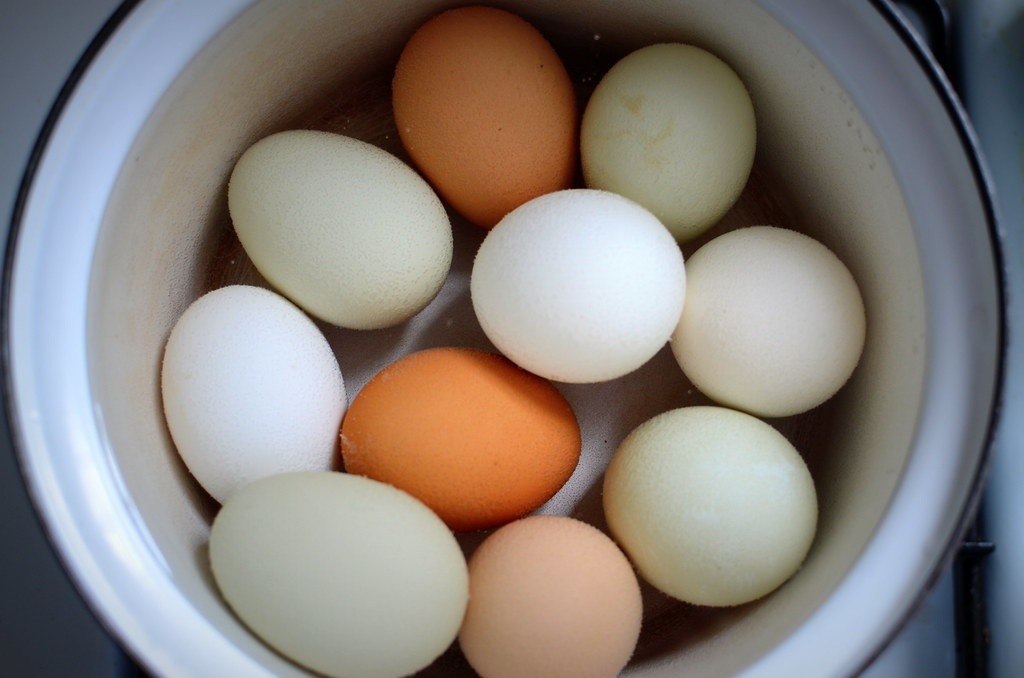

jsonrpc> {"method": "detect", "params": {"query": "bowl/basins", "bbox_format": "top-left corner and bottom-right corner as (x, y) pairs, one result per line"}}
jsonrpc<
(0, 0), (1009, 678)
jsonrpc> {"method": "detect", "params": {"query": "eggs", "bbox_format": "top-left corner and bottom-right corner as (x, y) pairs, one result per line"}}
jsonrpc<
(159, 2), (867, 678)
